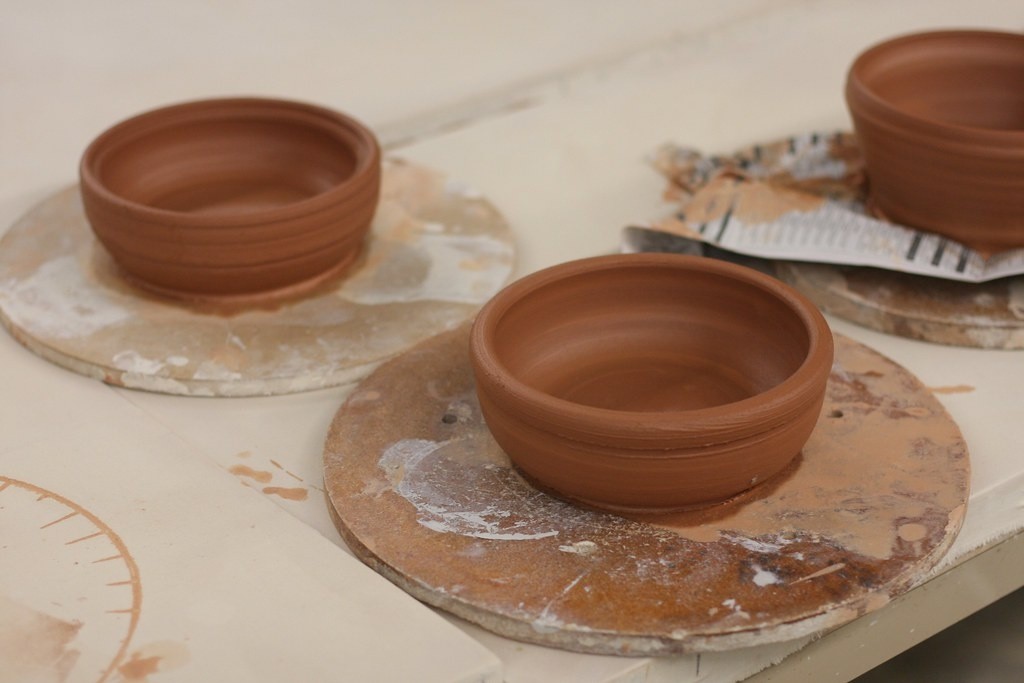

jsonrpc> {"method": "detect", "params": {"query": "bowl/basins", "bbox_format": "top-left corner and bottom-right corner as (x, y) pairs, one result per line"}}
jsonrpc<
(846, 30), (1024, 242)
(79, 96), (382, 294)
(470, 252), (836, 511)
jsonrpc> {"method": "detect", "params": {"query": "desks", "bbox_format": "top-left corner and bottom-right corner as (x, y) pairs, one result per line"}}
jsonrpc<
(0, 1), (1024, 683)
